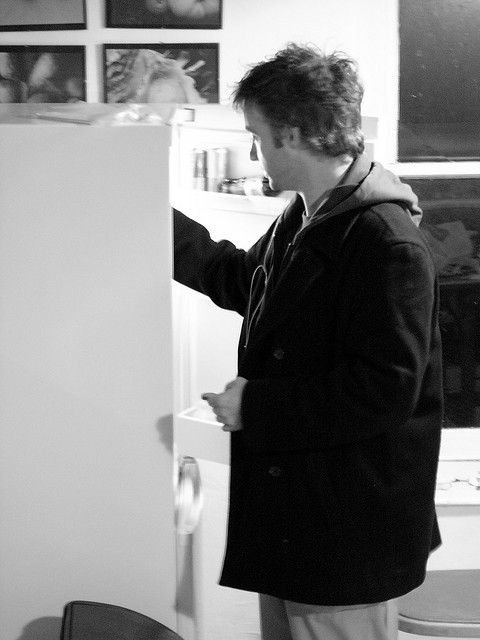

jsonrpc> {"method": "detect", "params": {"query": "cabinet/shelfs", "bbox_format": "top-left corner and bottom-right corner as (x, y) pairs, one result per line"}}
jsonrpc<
(0, 0), (239, 123)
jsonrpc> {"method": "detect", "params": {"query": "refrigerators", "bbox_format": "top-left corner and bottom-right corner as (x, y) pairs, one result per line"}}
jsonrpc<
(1, 107), (377, 640)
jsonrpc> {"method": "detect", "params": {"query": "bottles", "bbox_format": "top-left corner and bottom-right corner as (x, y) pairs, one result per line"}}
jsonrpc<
(189, 148), (205, 191)
(205, 147), (227, 194)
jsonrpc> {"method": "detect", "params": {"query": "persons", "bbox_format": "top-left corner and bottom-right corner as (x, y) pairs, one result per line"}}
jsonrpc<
(173, 45), (444, 640)
